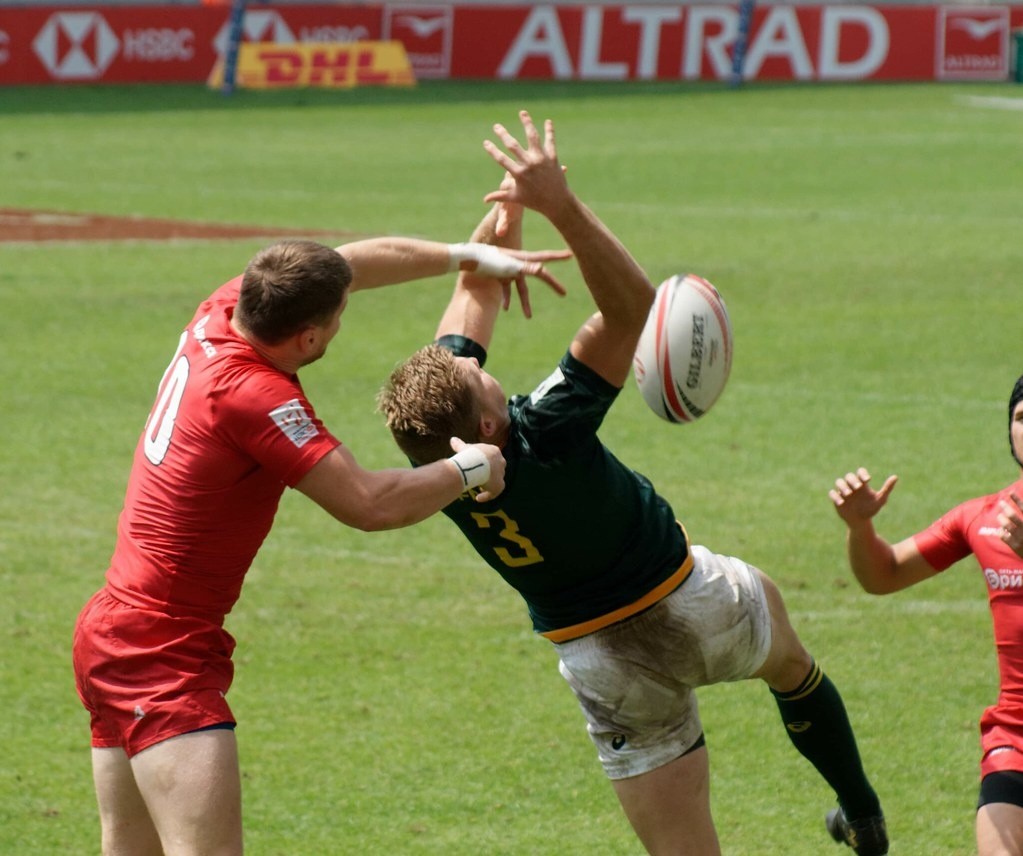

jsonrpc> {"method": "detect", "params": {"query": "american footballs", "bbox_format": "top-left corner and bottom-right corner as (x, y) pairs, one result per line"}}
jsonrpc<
(632, 271), (733, 425)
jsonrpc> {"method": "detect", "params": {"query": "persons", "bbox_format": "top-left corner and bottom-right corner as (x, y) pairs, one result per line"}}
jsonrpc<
(74, 236), (573, 856)
(374, 107), (893, 856)
(828, 375), (1023, 856)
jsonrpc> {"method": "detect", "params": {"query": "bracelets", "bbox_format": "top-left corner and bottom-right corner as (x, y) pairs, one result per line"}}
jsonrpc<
(448, 445), (492, 493)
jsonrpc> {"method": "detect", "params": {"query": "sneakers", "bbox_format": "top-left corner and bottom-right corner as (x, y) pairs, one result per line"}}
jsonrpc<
(825, 806), (889, 856)
